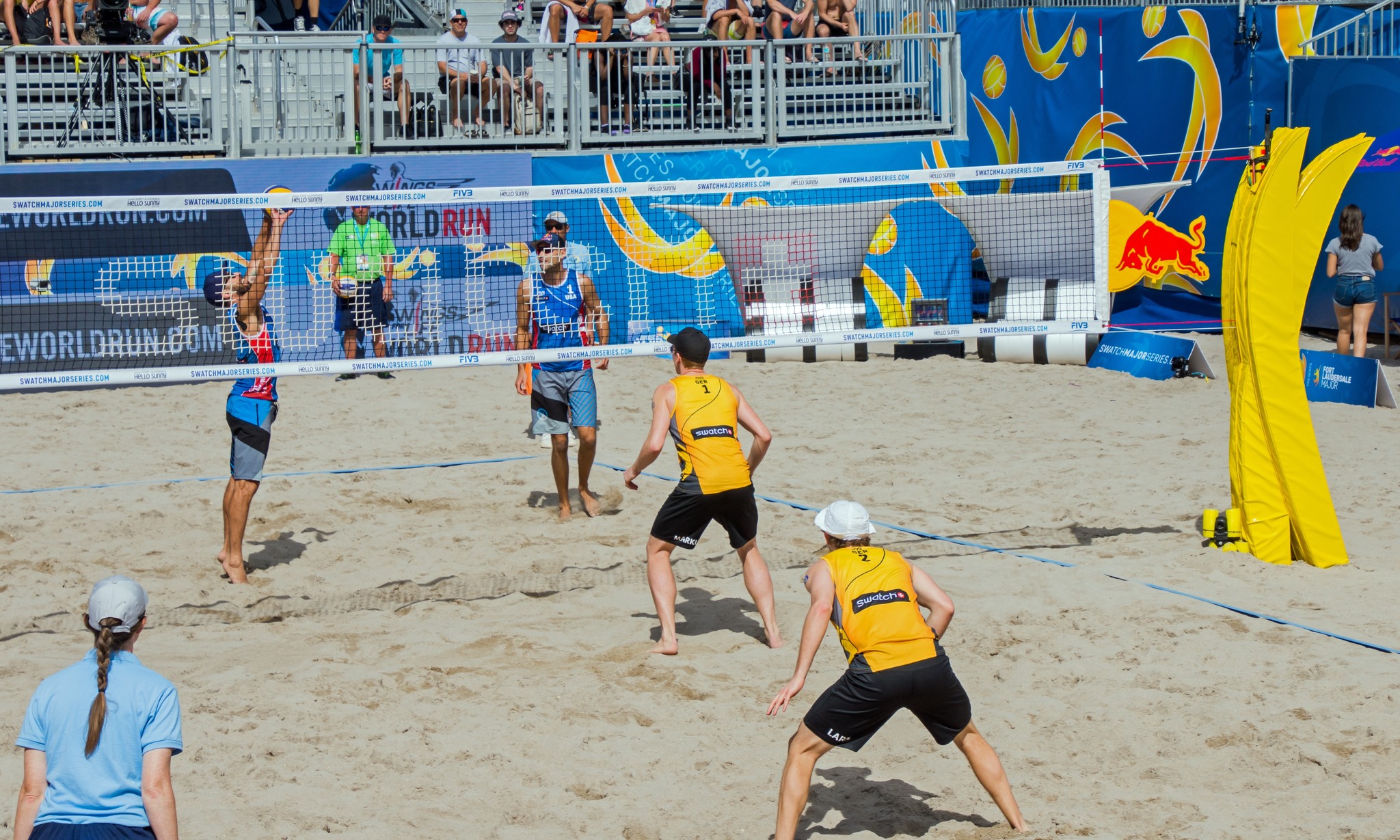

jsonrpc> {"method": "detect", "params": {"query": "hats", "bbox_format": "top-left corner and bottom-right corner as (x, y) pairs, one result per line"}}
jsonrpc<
(530, 212), (568, 250)
(667, 327), (711, 362)
(814, 500), (876, 541)
(373, 9), (519, 22)
(203, 261), (227, 307)
(88, 575), (149, 634)
(702, 34), (718, 49)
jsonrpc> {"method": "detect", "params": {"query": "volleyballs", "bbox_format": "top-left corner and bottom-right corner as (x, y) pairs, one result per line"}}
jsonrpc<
(337, 275), (359, 298)
(261, 184), (296, 219)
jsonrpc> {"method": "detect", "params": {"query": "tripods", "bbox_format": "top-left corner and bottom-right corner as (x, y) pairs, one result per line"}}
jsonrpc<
(56, 45), (194, 148)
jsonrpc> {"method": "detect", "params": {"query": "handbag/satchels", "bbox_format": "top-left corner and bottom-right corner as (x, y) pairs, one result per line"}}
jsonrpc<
(409, 92), (444, 137)
(120, 102), (180, 143)
(672, 67), (691, 92)
(632, 72), (646, 92)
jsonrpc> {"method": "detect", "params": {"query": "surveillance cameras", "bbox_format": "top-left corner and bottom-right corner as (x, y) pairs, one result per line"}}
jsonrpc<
(30, 278), (51, 292)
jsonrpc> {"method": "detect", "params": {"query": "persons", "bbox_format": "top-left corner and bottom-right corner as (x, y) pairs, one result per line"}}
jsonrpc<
(0, 1), (869, 585)
(11, 574), (183, 840)
(1325, 205), (1385, 358)
(758, 502), (1041, 839)
(624, 327), (783, 655)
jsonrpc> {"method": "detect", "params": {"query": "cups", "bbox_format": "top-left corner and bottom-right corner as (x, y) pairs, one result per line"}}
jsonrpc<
(470, 70), (478, 77)
(518, 76), (525, 86)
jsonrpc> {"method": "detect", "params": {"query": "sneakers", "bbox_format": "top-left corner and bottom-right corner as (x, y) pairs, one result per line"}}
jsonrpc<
(356, 118), (737, 138)
(515, 1), (684, 60)
(294, 16), (320, 36)
(377, 371), (396, 379)
(335, 373), (356, 382)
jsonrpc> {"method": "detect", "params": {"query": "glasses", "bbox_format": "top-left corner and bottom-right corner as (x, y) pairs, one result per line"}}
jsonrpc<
(614, 49), (627, 55)
(222, 271), (236, 291)
(670, 345), (677, 353)
(376, 17), (467, 31)
(543, 223), (563, 231)
(354, 205), (368, 208)
(536, 246), (553, 254)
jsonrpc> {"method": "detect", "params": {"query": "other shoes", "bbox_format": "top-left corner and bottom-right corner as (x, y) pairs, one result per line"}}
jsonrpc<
(697, 23), (707, 34)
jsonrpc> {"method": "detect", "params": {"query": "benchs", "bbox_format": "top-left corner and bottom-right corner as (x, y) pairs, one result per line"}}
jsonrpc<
(505, 0), (954, 133)
(0, 19), (212, 150)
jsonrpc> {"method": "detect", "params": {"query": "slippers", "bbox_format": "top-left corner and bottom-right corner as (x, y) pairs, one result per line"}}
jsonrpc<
(645, 57), (868, 83)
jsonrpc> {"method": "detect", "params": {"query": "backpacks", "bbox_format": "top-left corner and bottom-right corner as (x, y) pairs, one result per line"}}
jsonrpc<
(573, 29), (598, 60)
(510, 77), (542, 135)
(838, 39), (882, 76)
(13, 2), (209, 89)
(785, 44), (809, 78)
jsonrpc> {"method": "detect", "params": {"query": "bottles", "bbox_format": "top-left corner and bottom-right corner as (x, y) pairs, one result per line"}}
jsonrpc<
(354, 130), (360, 154)
(824, 44), (830, 62)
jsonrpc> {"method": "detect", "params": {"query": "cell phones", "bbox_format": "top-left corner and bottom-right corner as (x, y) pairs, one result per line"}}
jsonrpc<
(655, 5), (662, 8)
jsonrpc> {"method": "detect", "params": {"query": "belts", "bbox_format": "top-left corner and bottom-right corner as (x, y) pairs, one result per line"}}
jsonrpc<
(74, 0), (86, 3)
